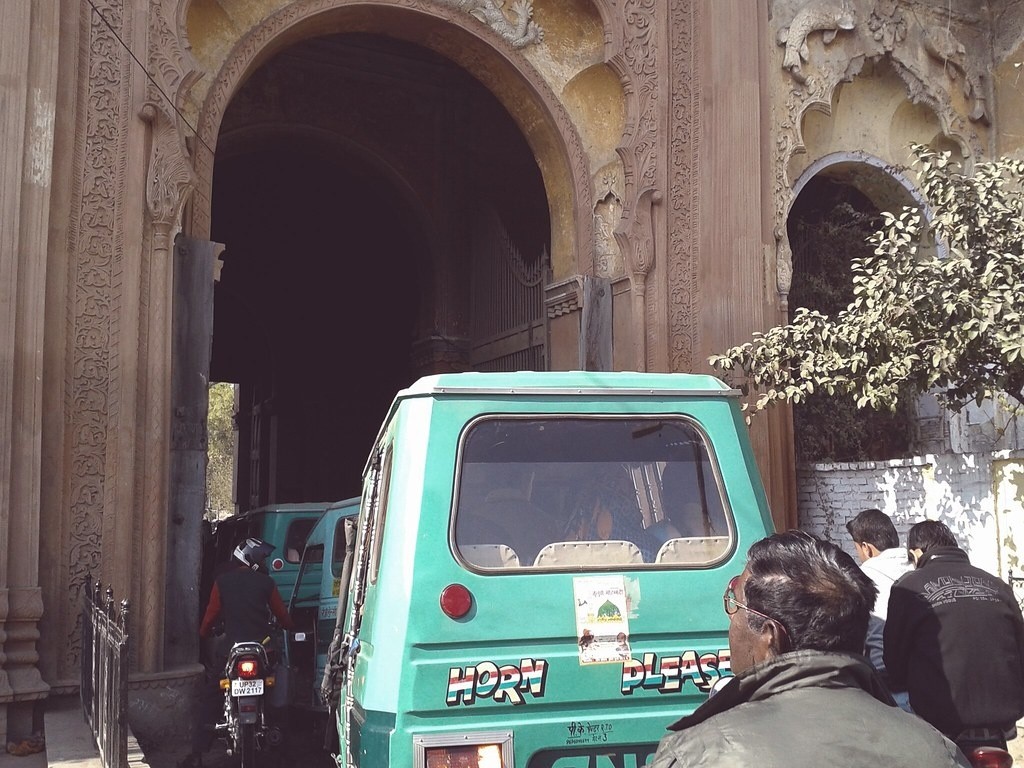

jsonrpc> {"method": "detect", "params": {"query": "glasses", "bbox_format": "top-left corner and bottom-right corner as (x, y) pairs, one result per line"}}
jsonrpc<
(722, 574), (793, 649)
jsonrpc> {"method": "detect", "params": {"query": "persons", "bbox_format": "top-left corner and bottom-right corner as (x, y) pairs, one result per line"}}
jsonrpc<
(646, 529), (972, 767)
(460, 450), (729, 568)
(883, 521), (1024, 741)
(207, 539), (291, 707)
(846, 511), (916, 715)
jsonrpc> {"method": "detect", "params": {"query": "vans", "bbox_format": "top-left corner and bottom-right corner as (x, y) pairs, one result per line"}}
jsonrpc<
(284, 497), (360, 712)
(325, 370), (776, 767)
(212, 501), (340, 613)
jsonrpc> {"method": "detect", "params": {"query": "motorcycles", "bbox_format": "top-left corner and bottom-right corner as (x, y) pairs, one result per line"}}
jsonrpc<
(210, 619), (282, 767)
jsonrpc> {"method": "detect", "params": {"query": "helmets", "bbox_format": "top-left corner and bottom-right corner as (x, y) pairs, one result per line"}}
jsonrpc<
(233, 537), (276, 571)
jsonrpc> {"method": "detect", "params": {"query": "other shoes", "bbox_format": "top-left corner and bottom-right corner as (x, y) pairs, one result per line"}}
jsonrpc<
(179, 754), (202, 768)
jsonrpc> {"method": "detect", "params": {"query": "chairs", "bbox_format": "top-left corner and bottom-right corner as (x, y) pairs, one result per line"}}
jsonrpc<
(458, 544), (519, 567)
(535, 541), (644, 567)
(656, 537), (727, 564)
